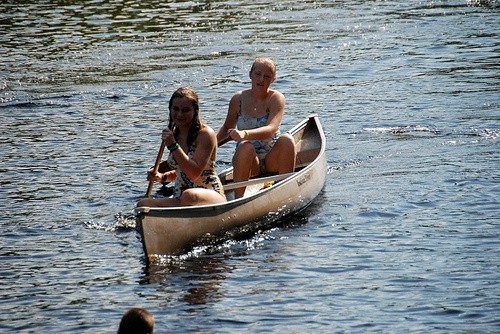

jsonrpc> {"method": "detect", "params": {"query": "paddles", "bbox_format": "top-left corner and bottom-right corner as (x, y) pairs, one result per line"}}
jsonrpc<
(148, 135), (233, 187)
(142, 121), (175, 199)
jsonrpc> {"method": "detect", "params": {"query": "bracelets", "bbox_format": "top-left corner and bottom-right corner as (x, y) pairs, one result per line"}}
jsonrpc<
(167, 142), (180, 153)
(137, 87), (227, 207)
(243, 130), (249, 139)
(162, 173), (168, 181)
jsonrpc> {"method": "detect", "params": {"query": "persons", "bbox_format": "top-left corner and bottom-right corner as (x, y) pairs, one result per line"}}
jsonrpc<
(215, 57), (296, 199)
(118, 309), (155, 334)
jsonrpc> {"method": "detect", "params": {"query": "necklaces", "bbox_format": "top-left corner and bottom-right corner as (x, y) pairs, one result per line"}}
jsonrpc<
(253, 106), (258, 110)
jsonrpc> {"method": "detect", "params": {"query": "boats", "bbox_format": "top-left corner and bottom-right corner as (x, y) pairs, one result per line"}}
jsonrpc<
(134, 113), (326, 257)
